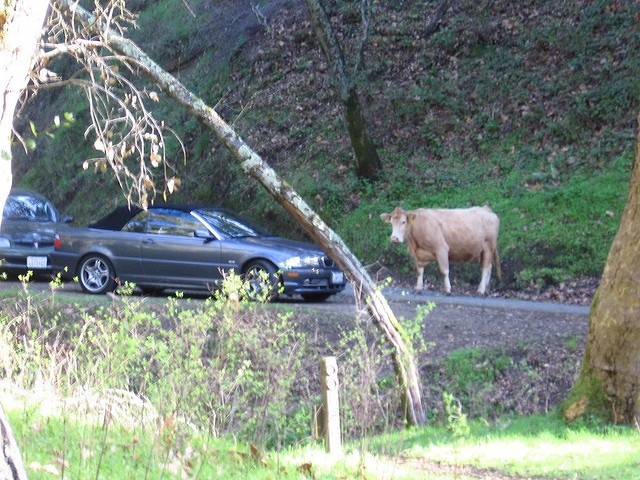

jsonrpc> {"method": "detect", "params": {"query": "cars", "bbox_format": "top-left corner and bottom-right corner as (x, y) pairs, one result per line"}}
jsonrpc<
(49, 203), (347, 303)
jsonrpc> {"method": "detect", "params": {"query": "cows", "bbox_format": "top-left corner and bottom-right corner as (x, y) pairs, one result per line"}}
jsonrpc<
(379, 205), (501, 297)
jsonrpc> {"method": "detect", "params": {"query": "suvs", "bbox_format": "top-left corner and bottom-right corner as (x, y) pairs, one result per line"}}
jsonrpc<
(0, 189), (75, 286)
